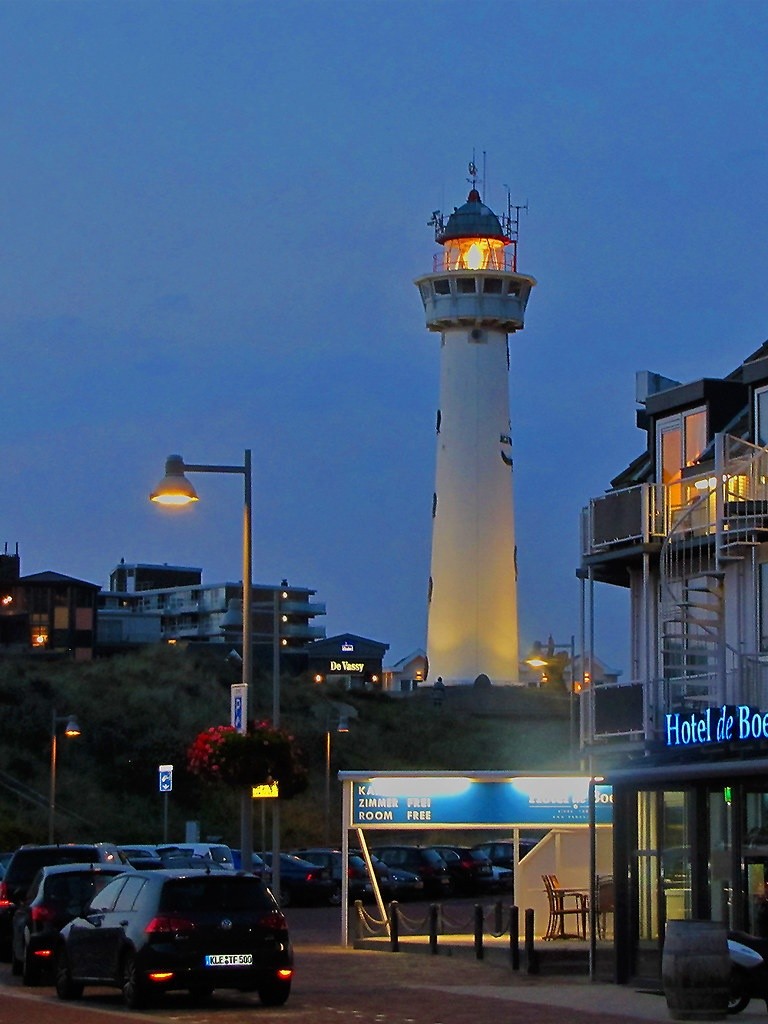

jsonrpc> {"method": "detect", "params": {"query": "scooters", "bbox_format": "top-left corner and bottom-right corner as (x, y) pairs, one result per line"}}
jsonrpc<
(724, 930), (768, 1014)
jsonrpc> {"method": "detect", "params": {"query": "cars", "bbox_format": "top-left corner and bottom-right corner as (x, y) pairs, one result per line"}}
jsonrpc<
(0, 837), (547, 911)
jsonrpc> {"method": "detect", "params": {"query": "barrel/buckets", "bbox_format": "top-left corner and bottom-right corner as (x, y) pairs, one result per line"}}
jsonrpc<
(661, 920), (732, 1019)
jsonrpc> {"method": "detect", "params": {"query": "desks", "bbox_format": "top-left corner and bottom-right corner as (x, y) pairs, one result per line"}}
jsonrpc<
(597, 873), (614, 941)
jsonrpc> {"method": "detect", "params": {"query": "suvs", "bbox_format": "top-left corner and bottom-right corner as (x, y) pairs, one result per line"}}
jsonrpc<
(52, 868), (294, 1006)
(10, 861), (136, 988)
(0, 843), (127, 963)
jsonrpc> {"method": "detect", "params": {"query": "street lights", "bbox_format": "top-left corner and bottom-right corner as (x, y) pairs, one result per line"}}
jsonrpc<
(151, 447), (253, 872)
(46, 706), (82, 847)
(522, 633), (576, 771)
(323, 711), (349, 849)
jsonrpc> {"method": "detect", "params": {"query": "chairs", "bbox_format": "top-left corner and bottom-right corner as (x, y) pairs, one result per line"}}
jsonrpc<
(540, 873), (590, 941)
(549, 874), (602, 940)
